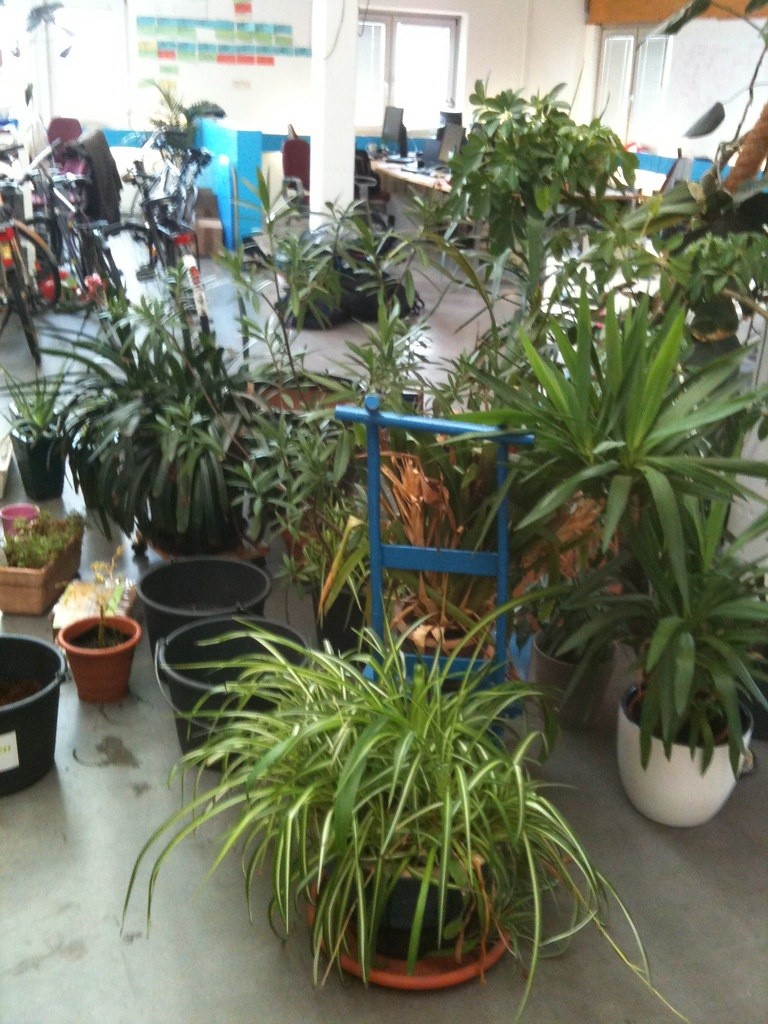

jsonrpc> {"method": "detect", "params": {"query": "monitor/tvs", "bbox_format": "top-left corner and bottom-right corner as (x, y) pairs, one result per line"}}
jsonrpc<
(382, 106), (414, 163)
(438, 111), (466, 174)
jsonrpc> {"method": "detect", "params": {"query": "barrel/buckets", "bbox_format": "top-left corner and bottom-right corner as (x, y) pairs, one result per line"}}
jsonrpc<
(138, 559), (270, 672)
(0, 633), (67, 797)
(152, 617), (308, 771)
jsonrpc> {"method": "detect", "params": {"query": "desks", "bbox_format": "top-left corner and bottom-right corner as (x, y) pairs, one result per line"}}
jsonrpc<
(608, 166), (667, 238)
(370, 151), (638, 249)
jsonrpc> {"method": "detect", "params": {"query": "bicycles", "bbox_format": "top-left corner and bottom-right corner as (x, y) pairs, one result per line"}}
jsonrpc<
(1, 124), (213, 363)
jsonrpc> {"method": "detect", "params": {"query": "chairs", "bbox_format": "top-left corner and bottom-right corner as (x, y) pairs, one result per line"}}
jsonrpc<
(46, 118), (82, 167)
(33, 125), (122, 204)
(281, 140), (313, 210)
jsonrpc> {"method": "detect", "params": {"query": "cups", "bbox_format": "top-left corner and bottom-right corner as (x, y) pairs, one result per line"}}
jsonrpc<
(0, 503), (39, 543)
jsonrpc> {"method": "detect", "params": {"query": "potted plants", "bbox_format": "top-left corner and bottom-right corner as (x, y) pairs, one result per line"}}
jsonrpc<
(0, 0), (768, 1024)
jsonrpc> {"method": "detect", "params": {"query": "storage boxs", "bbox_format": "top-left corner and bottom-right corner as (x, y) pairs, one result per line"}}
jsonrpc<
(195, 188), (223, 256)
